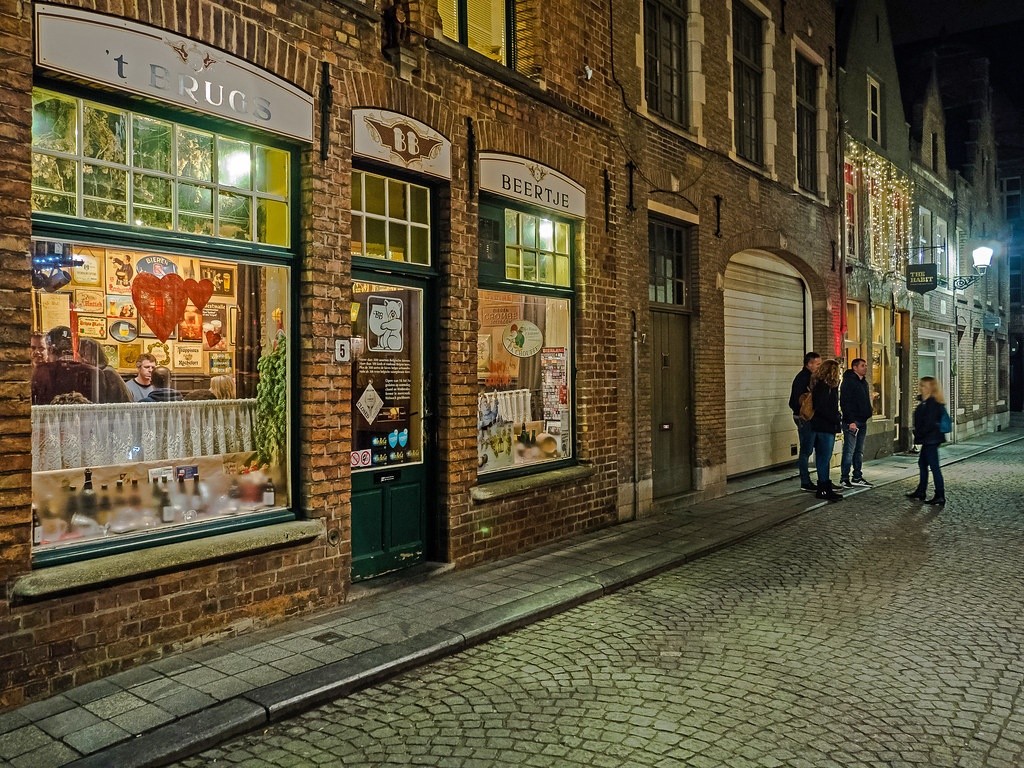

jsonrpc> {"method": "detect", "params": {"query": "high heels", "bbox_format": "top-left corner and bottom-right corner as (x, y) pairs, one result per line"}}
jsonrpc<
(905, 489), (926, 501)
(924, 496), (946, 506)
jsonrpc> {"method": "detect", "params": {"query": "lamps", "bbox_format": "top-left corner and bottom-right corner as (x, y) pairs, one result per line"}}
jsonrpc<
(953, 246), (994, 291)
(43, 271), (72, 292)
(32, 273), (48, 289)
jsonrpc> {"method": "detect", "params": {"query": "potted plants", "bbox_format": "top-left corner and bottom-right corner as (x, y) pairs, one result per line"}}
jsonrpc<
(937, 274), (951, 288)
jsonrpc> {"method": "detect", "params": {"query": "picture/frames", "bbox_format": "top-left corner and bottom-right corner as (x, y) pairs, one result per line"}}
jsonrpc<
(228, 305), (237, 345)
(77, 315), (108, 339)
(37, 289), (74, 334)
(200, 265), (235, 297)
(137, 309), (177, 340)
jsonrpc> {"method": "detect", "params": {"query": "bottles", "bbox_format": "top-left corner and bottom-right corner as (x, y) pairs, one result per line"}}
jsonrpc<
(128, 478), (143, 512)
(98, 484), (112, 526)
(176, 473), (188, 495)
(160, 474), (174, 523)
(150, 476), (161, 517)
(517, 422), (536, 448)
(32, 504), (42, 545)
(81, 467), (97, 526)
(191, 473), (202, 513)
(67, 486), (80, 532)
(112, 480), (128, 520)
(262, 476), (276, 506)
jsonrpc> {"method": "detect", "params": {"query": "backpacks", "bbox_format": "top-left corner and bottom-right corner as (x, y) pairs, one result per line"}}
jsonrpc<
(936, 404), (952, 433)
(799, 388), (831, 421)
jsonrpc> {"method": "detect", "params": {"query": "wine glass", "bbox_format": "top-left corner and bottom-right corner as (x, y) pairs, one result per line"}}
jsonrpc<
(172, 504), (197, 522)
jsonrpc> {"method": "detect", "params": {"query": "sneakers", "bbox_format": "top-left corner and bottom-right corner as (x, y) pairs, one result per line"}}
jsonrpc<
(840, 479), (854, 489)
(816, 489), (843, 501)
(832, 483), (843, 491)
(851, 478), (873, 487)
(801, 483), (817, 492)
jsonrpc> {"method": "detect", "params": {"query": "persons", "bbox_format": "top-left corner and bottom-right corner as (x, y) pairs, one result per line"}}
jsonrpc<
(788, 352), (874, 502)
(30, 327), (236, 406)
(904, 375), (953, 507)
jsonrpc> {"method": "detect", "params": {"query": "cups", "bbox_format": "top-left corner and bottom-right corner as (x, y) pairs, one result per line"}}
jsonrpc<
(119, 323), (129, 337)
(44, 518), (67, 541)
(109, 299), (116, 316)
(222, 273), (231, 292)
